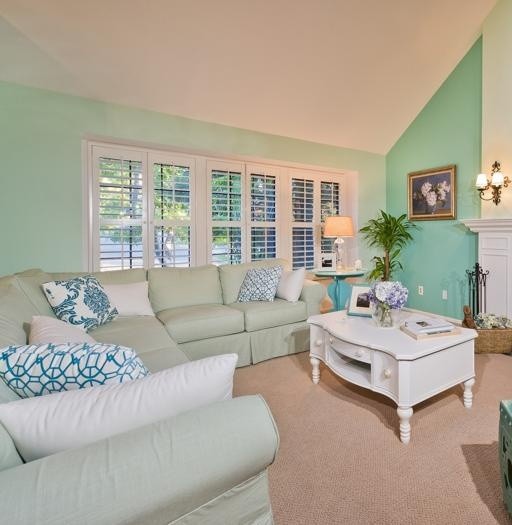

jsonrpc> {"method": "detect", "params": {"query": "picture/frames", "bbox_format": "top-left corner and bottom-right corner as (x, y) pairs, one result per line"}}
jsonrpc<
(407, 164), (457, 221)
(347, 283), (376, 317)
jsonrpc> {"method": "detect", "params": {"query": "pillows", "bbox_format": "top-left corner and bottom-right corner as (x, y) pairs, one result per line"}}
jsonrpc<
(102, 282), (156, 315)
(277, 267), (305, 302)
(239, 266), (283, 303)
(42, 276), (117, 328)
(0, 353), (239, 461)
(28, 314), (96, 345)
(1, 344), (147, 398)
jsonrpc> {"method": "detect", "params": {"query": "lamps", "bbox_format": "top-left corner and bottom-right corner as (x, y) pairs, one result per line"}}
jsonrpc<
(324, 216), (354, 272)
(475, 161), (512, 204)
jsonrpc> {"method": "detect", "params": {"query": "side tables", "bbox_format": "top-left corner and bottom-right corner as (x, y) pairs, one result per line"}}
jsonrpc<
(316, 269), (364, 308)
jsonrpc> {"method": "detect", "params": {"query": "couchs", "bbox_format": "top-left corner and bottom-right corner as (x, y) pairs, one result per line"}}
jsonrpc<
(1, 261), (326, 525)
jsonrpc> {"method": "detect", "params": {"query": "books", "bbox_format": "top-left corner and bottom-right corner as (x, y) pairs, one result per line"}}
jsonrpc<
(400, 319), (459, 342)
(404, 316), (454, 334)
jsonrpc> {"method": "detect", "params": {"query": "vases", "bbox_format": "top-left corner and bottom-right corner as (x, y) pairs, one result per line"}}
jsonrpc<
(373, 305), (397, 327)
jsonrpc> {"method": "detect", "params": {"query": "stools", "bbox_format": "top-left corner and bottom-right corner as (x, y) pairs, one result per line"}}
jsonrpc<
(498, 399), (511, 519)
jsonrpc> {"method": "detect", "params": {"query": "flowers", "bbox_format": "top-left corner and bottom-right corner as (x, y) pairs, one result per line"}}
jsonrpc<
(368, 281), (409, 308)
